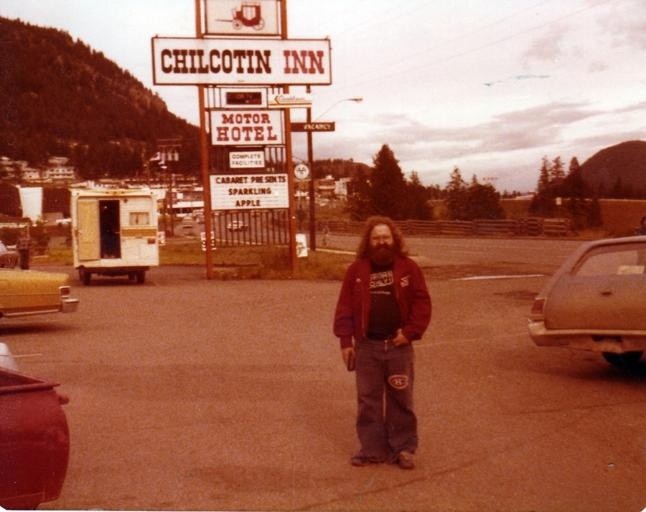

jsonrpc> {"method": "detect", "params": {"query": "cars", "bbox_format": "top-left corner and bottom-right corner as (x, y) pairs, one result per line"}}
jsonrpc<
(0, 267), (78, 322)
(180, 207), (205, 229)
(228, 220), (249, 233)
(526, 235), (646, 368)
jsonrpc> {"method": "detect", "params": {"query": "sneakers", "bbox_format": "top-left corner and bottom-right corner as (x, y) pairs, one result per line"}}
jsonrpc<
(398, 452), (414, 470)
(351, 457), (371, 465)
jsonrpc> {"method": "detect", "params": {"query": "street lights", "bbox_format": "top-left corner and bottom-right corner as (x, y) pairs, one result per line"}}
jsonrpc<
(312, 97), (363, 122)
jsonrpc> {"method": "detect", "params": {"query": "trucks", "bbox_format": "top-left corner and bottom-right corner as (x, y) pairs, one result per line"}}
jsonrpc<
(70, 188), (161, 285)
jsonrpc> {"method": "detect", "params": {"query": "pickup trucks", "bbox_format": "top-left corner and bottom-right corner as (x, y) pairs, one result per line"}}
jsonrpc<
(1, 365), (73, 511)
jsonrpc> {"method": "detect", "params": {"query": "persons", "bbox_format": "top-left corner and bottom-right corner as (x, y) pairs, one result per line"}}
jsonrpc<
(634, 216), (646, 234)
(295, 205), (307, 230)
(16, 230), (32, 269)
(333, 216), (432, 469)
(319, 223), (330, 249)
